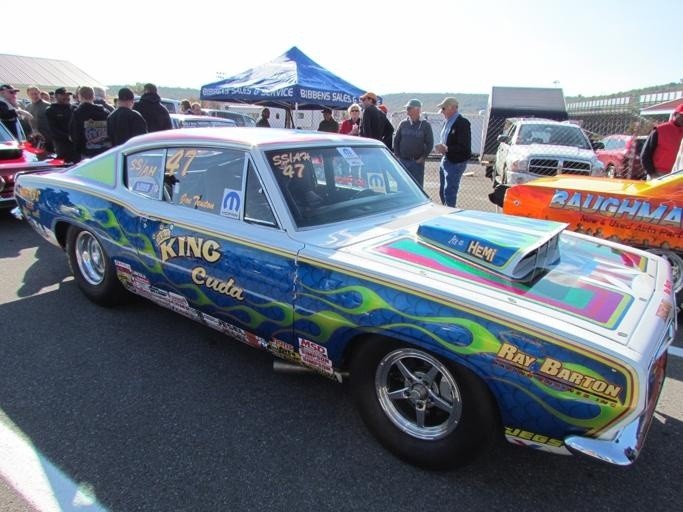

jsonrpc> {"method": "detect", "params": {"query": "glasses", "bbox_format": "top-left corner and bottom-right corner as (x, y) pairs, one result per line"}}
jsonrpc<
(351, 110), (359, 112)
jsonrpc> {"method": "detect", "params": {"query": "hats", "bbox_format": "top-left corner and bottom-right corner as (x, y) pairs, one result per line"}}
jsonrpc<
(675, 104), (683, 114)
(379, 105), (387, 113)
(55, 88), (72, 95)
(360, 92), (377, 101)
(322, 109), (332, 113)
(404, 99), (422, 106)
(0, 85), (19, 92)
(437, 97), (458, 107)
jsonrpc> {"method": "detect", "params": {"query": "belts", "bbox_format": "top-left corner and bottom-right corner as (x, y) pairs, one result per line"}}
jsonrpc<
(400, 156), (420, 161)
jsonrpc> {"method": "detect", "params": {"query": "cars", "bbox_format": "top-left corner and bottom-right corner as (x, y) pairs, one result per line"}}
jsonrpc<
(0, 119), (66, 215)
(592, 131), (651, 179)
(113, 95), (260, 130)
(498, 167), (682, 313)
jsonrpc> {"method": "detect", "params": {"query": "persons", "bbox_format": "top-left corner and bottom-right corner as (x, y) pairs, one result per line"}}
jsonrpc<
(0, 83), (173, 166)
(359, 91), (386, 141)
(434, 97), (471, 208)
(255, 108), (271, 127)
(378, 105), (394, 150)
(639, 104), (683, 181)
(317, 108), (338, 132)
(338, 104), (363, 188)
(179, 100), (192, 114)
(392, 98), (434, 191)
(191, 103), (208, 115)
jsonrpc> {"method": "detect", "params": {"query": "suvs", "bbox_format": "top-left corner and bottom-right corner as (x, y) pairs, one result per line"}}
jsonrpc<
(493, 115), (604, 197)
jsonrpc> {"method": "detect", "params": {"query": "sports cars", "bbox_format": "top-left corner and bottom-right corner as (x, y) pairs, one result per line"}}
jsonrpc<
(12, 121), (676, 470)
